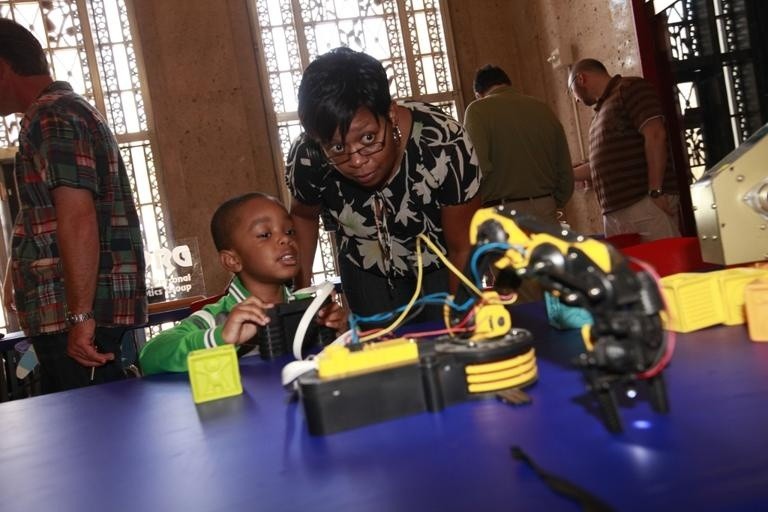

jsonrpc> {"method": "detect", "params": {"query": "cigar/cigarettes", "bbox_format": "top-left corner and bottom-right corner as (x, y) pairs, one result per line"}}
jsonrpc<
(88, 346), (97, 382)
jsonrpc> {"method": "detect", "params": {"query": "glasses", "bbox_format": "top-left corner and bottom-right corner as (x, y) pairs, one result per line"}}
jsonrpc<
(319, 116), (389, 168)
(564, 76), (577, 97)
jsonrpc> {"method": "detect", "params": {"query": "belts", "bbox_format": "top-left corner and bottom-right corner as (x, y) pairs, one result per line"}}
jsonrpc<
(481, 192), (552, 208)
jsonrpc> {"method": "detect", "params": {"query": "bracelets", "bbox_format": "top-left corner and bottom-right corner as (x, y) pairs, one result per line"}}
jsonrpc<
(65, 311), (95, 325)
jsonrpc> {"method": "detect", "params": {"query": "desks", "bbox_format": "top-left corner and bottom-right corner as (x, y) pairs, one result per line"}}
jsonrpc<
(0, 298), (767, 511)
(0, 292), (209, 400)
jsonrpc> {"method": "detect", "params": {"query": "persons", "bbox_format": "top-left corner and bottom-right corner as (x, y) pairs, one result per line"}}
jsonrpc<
(567, 59), (683, 243)
(136, 191), (346, 376)
(282, 45), (483, 328)
(0, 16), (148, 391)
(460, 62), (573, 238)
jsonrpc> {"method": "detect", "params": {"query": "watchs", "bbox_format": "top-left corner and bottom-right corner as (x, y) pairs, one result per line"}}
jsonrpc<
(649, 187), (662, 199)
